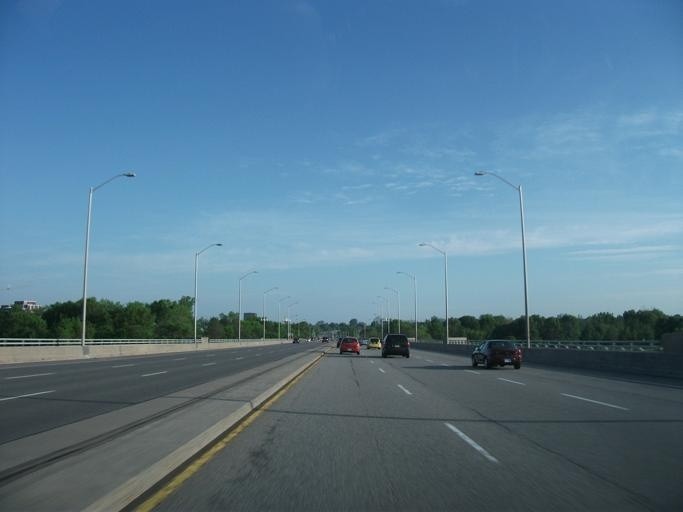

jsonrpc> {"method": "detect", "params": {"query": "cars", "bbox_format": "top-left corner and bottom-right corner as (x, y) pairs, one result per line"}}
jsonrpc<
(293, 337), (321, 344)
(471, 340), (522, 369)
(322, 337), (329, 343)
(337, 334), (410, 358)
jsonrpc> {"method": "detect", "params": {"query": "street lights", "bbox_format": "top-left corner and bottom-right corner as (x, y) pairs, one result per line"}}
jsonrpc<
(373, 271), (418, 343)
(262, 286), (299, 341)
(474, 170), (531, 349)
(82, 172), (136, 346)
(419, 243), (449, 344)
(194, 240), (224, 344)
(238, 270), (258, 340)
